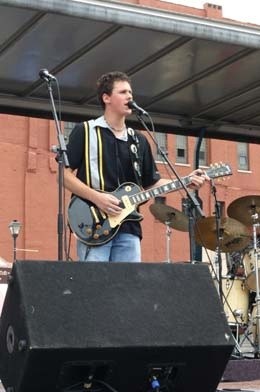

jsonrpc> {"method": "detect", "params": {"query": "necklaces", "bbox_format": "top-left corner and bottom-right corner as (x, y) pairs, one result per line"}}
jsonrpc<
(105, 117), (125, 131)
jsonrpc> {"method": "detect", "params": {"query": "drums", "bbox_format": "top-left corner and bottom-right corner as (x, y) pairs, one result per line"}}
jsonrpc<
(250, 298), (260, 352)
(239, 244), (260, 292)
(214, 274), (249, 327)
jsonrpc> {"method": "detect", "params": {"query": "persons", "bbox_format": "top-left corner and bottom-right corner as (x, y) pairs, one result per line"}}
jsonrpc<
(54, 72), (211, 263)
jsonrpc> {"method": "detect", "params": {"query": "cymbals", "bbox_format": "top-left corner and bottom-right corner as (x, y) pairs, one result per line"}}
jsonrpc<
(194, 215), (251, 253)
(148, 202), (192, 233)
(226, 194), (260, 225)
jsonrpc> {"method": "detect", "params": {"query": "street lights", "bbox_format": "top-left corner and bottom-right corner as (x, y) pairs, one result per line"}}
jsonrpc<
(7, 219), (21, 260)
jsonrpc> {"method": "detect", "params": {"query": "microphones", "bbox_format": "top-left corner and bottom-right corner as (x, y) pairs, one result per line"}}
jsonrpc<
(128, 101), (148, 115)
(39, 69), (57, 82)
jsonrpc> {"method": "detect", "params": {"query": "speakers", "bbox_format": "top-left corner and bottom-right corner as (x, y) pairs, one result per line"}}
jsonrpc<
(0, 260), (237, 392)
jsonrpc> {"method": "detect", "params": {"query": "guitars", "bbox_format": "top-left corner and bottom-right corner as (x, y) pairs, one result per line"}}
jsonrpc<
(67, 159), (235, 248)
